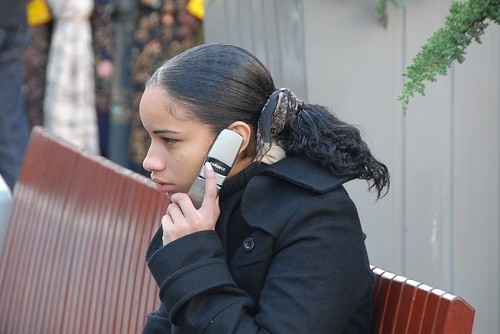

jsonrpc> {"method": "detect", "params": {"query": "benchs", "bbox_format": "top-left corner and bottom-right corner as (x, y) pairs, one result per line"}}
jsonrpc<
(1, 121), (477, 334)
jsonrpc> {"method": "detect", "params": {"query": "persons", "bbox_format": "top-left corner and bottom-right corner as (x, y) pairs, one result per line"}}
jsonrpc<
(138, 44), (391, 334)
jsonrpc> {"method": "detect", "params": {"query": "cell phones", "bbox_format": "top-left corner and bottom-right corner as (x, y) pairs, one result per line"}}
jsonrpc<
(188, 128), (245, 210)
